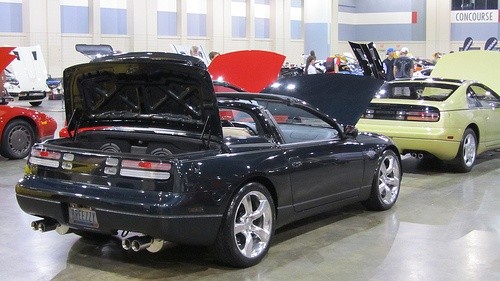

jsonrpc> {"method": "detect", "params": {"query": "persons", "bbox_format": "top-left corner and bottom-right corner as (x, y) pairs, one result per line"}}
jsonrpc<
(209, 52), (220, 61)
(190, 45), (205, 65)
(381, 48), (414, 81)
(304, 50), (317, 75)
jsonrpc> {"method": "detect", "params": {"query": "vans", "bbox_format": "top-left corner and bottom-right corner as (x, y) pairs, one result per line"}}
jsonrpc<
(4, 45), (50, 107)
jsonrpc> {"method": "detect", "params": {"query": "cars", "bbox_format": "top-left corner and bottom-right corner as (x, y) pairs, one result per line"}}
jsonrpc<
(0, 47), (57, 161)
(15, 51), (403, 269)
(59, 50), (302, 139)
(354, 50), (500, 173)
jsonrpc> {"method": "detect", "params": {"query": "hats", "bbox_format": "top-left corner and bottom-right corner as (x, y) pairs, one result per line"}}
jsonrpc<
(386, 48), (394, 55)
(401, 47), (409, 53)
